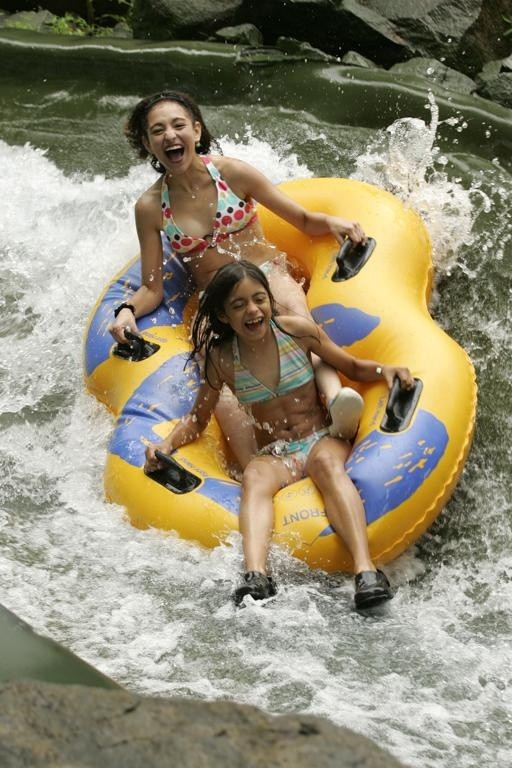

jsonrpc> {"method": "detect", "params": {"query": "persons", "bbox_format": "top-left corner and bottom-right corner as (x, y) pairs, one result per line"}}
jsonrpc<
(106, 88), (368, 473)
(142, 260), (417, 608)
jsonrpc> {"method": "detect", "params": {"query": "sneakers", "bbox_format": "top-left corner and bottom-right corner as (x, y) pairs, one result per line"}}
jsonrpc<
(354, 568), (394, 610)
(329, 387), (364, 442)
(232, 571), (279, 606)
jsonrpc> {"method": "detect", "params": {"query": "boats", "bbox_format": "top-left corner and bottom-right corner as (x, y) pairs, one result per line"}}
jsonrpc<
(82, 178), (478, 577)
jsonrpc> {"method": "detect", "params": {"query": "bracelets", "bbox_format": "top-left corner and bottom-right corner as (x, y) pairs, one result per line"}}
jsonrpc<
(115, 303), (135, 318)
